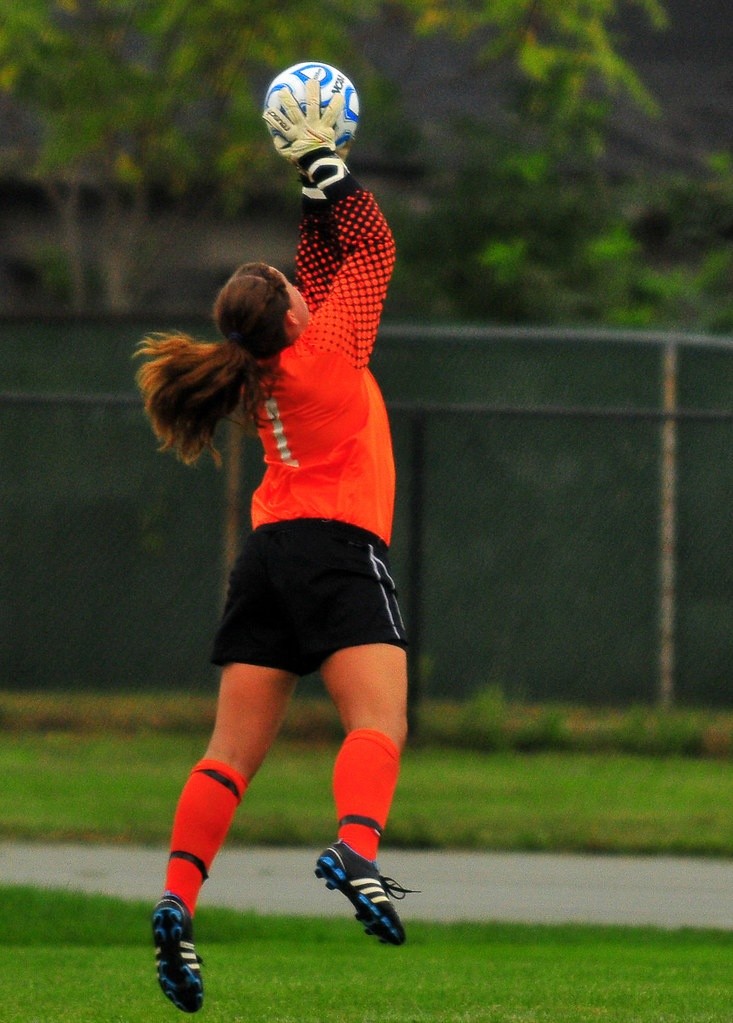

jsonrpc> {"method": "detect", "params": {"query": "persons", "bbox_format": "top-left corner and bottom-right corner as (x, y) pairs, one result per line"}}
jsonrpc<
(130, 78), (409, 1013)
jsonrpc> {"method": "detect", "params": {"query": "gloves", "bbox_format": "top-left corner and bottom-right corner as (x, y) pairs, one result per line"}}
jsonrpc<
(262, 78), (364, 206)
(298, 167), (332, 214)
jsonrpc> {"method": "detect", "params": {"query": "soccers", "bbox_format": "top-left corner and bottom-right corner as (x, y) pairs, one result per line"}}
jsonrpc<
(264, 62), (361, 157)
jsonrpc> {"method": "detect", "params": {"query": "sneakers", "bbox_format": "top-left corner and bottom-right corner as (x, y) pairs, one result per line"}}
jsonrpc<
(314, 838), (421, 946)
(151, 891), (207, 1014)
